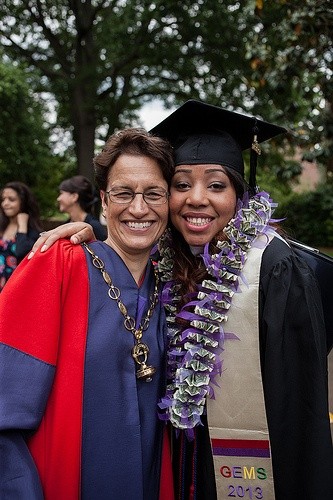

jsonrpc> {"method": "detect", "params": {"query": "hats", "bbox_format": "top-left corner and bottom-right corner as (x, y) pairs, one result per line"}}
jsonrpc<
(144, 99), (288, 199)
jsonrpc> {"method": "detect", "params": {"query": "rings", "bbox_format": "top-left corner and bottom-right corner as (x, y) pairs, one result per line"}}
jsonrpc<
(39, 232), (48, 236)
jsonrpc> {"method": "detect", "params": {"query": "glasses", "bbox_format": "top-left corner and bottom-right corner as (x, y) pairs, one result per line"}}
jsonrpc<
(104, 185), (171, 205)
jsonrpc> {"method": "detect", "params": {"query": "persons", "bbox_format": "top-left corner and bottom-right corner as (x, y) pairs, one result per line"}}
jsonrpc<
(28, 100), (333, 500)
(57, 175), (108, 242)
(0, 128), (196, 500)
(0, 183), (41, 293)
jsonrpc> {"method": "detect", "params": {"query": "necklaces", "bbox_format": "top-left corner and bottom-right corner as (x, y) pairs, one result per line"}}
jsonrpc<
(81, 241), (160, 382)
